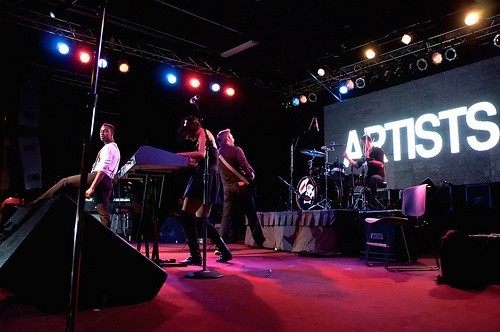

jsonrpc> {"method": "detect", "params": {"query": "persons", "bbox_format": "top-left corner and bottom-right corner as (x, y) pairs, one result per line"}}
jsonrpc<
(15, 123), (121, 228)
(213, 128), (266, 254)
(175, 116), (233, 265)
(342, 135), (386, 210)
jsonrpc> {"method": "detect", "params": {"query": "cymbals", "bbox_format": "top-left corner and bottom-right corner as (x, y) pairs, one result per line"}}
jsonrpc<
(300, 149), (326, 157)
(324, 143), (347, 147)
(352, 158), (376, 162)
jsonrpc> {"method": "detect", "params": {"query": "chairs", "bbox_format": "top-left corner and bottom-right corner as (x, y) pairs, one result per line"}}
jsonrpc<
(365, 183), (442, 273)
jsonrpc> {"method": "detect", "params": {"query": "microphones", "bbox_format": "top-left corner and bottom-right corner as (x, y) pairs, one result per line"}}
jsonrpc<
(189, 95), (200, 104)
(299, 195), (305, 199)
(315, 118), (320, 131)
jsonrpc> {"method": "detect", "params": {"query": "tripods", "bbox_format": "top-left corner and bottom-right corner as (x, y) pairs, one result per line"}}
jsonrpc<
(307, 150), (337, 211)
(340, 133), (385, 211)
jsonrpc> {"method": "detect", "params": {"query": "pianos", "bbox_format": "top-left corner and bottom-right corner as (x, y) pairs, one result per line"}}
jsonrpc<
(117, 144), (190, 268)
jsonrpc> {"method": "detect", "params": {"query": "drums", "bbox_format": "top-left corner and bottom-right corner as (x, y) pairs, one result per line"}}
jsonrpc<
(325, 161), (346, 177)
(295, 175), (342, 212)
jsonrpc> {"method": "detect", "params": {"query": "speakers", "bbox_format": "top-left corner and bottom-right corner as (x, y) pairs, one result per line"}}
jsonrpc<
(0, 193), (188, 316)
(12, 97), (43, 193)
(366, 178), (500, 260)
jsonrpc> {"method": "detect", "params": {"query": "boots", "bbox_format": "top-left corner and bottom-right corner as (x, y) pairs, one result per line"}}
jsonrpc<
(196, 216), (232, 262)
(179, 209), (202, 265)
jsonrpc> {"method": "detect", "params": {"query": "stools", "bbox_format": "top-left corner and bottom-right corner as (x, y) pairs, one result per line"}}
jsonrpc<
(363, 182), (388, 210)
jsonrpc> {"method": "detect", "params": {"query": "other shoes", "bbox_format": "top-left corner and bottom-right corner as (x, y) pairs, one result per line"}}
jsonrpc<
(257, 236), (266, 246)
(15, 204), (32, 209)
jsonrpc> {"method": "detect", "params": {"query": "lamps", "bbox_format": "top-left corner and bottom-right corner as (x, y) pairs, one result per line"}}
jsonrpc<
(493, 33), (500, 47)
(282, 30), (460, 108)
